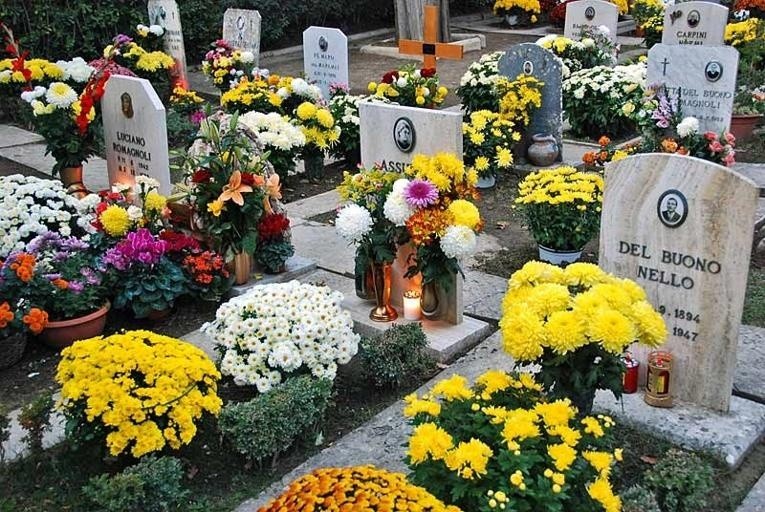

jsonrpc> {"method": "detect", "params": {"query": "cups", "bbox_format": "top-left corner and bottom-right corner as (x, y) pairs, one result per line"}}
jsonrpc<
(646, 348), (675, 406)
(623, 359), (640, 393)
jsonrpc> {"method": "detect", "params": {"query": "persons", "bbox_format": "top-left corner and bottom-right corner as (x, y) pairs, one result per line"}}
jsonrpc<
(586, 8), (593, 19)
(708, 64), (719, 78)
(122, 93), (134, 117)
(236, 16), (244, 30)
(396, 122), (411, 147)
(663, 198), (681, 222)
(524, 62), (531, 72)
(688, 13), (697, 26)
(159, 6), (166, 19)
(319, 35), (327, 50)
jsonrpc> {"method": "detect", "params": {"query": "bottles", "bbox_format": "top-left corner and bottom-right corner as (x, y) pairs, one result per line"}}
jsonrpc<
(527, 132), (558, 166)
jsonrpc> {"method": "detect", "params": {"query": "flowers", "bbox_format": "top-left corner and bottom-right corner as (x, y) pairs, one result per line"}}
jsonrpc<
(0, 0), (765, 512)
